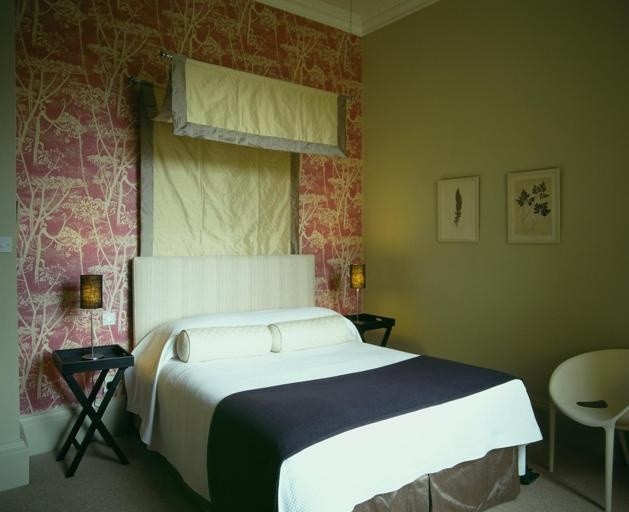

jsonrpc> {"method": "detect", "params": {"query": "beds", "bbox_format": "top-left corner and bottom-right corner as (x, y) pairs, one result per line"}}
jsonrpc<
(132, 253), (544, 511)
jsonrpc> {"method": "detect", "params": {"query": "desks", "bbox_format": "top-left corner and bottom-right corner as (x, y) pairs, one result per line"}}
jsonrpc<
(51, 344), (134, 478)
(344, 312), (395, 346)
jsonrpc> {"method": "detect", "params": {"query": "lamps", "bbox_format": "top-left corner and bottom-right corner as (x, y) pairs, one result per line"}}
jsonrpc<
(349, 264), (365, 326)
(80, 274), (103, 360)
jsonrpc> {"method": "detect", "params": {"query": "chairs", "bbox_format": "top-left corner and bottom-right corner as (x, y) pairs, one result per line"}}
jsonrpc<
(546, 348), (629, 512)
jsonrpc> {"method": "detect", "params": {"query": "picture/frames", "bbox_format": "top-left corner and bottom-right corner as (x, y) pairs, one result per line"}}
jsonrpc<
(435, 175), (480, 243)
(506, 167), (561, 245)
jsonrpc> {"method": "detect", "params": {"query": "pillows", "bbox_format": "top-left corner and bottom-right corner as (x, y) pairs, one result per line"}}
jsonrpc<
(154, 305), (361, 363)
